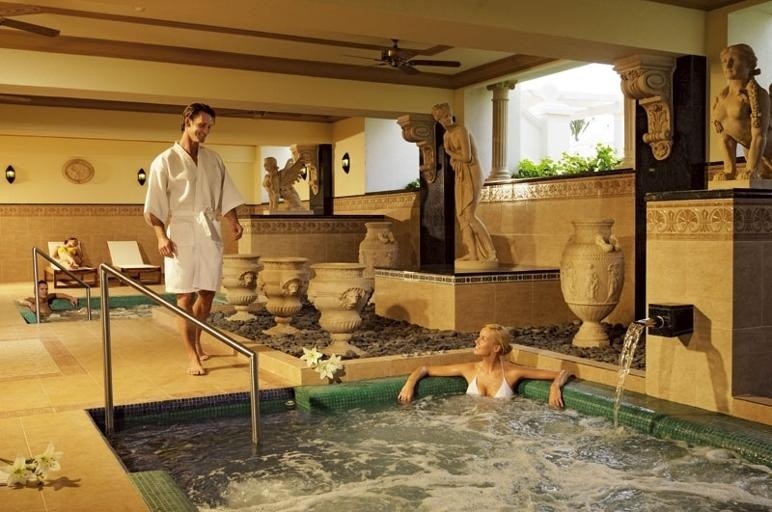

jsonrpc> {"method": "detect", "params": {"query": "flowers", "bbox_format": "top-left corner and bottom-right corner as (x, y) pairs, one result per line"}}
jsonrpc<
(299, 346), (343, 380)
(0, 443), (63, 487)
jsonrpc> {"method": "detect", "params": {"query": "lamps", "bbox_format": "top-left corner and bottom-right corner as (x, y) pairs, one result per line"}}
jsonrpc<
(6, 165), (15, 185)
(342, 152), (350, 175)
(301, 163), (307, 180)
(138, 168), (146, 185)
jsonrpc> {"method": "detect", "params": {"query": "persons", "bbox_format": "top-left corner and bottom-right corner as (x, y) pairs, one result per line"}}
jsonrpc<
(262, 153), (302, 211)
(54, 237), (84, 269)
(395, 323), (573, 409)
(17, 278), (80, 321)
(430, 103), (499, 261)
(143, 102), (244, 377)
(709, 43), (772, 180)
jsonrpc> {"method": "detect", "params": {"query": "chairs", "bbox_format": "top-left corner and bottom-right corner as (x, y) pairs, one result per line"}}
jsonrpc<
(106, 240), (162, 288)
(45, 240), (100, 288)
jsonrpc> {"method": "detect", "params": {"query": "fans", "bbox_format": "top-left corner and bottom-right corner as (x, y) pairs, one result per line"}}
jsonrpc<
(339, 38), (461, 76)
